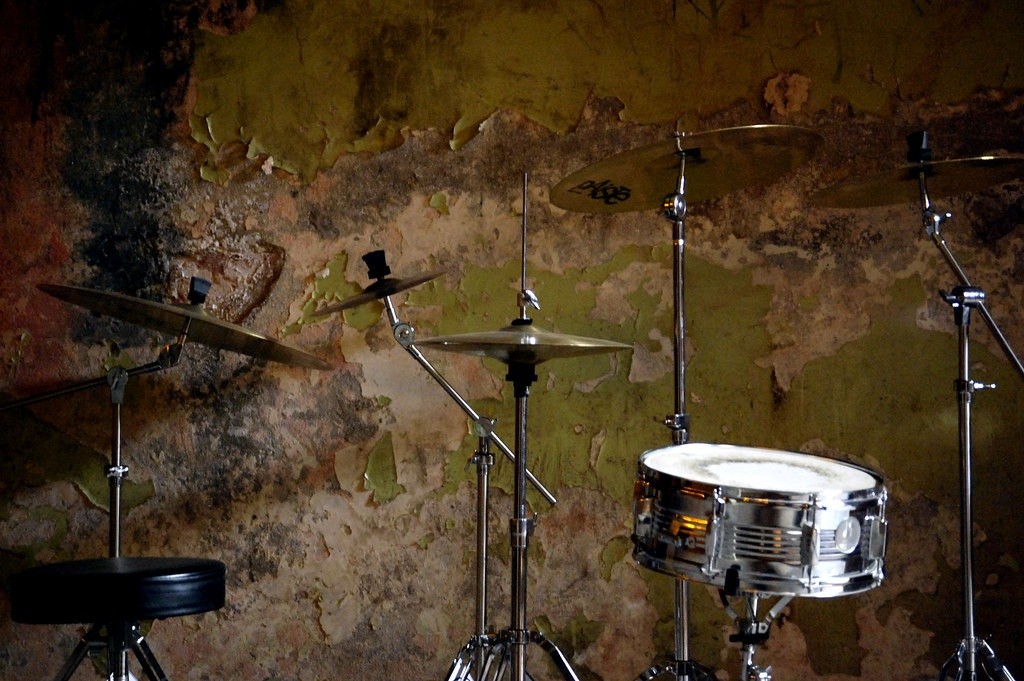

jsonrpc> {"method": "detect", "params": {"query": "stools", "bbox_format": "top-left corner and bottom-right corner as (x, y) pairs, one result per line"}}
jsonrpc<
(9, 555), (226, 681)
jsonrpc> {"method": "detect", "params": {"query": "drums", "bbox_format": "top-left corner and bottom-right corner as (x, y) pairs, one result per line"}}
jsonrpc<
(631, 443), (891, 596)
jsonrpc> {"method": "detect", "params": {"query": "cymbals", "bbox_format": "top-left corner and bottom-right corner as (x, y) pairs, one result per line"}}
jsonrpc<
(403, 325), (636, 364)
(309, 269), (449, 318)
(34, 280), (335, 373)
(547, 122), (830, 217)
(807, 155), (1023, 210)
